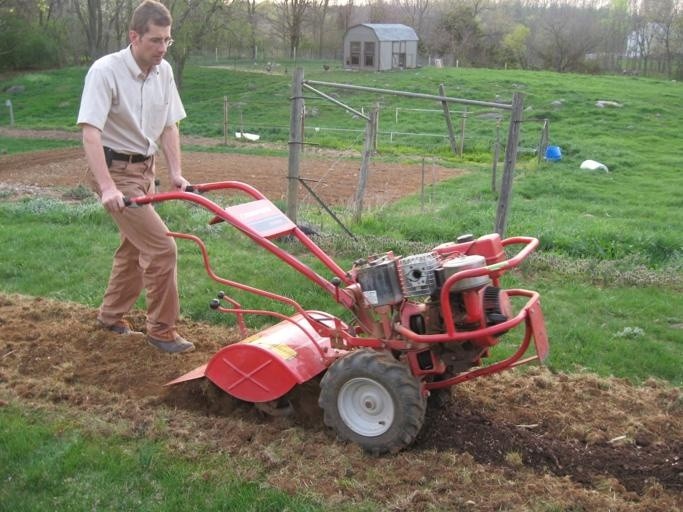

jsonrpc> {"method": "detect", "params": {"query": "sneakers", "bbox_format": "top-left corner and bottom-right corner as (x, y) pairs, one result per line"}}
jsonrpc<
(96, 320), (196, 353)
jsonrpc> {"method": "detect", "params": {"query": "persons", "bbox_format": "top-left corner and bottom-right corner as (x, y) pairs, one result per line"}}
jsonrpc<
(72, 1), (197, 354)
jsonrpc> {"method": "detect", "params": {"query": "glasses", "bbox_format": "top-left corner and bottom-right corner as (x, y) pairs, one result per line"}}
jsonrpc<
(149, 36), (173, 46)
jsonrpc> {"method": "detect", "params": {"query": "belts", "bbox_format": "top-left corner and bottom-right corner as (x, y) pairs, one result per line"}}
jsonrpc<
(111, 153), (148, 162)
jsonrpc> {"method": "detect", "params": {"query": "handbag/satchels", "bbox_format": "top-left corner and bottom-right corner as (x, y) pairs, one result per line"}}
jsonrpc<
(104, 146), (111, 168)
